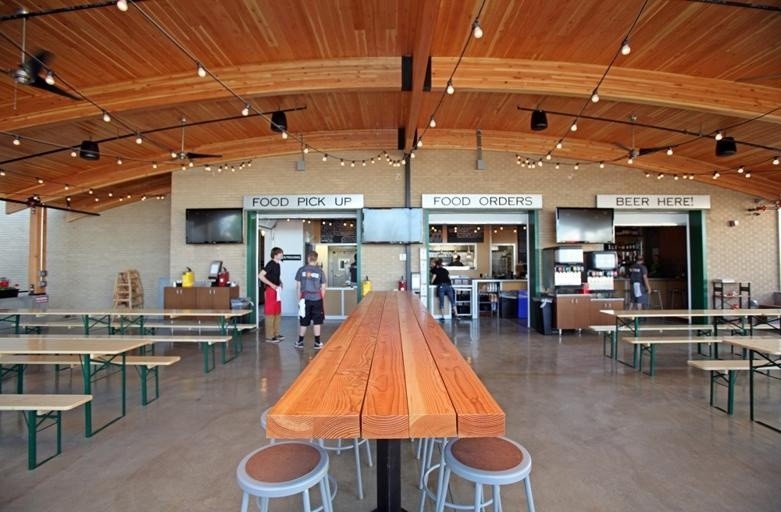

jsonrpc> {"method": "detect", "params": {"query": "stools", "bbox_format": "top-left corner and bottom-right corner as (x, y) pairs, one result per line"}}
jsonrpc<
(411, 436), (535, 511)
(111, 266), (147, 331)
(605, 289), (618, 298)
(646, 289), (666, 320)
(623, 288), (638, 311)
(236, 406), (374, 512)
(668, 287), (682, 320)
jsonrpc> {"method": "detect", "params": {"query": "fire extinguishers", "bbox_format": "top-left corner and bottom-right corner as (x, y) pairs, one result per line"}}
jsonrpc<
(399, 276), (407, 290)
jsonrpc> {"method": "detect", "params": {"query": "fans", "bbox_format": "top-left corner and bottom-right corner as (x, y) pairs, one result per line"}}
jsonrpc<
(0, 6), (86, 106)
(609, 108), (673, 164)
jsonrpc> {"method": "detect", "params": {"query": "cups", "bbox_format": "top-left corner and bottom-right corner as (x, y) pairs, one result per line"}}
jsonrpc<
(176, 279), (182, 286)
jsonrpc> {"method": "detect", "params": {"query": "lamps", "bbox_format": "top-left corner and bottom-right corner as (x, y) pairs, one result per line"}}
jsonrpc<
(716, 128), (740, 161)
(527, 103), (552, 132)
(78, 134), (103, 162)
(267, 103), (292, 136)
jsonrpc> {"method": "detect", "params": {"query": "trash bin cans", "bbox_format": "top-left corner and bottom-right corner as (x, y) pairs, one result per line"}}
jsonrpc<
(499, 290), (528, 318)
(230, 296), (251, 333)
(532, 296), (552, 335)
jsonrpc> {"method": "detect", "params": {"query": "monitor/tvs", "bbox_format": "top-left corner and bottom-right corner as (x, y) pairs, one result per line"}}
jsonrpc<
(556, 206), (615, 244)
(362, 207), (423, 244)
(208, 260), (222, 278)
(186, 207), (243, 244)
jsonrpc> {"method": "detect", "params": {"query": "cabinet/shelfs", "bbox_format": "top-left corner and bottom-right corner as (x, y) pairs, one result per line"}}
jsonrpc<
(197, 286), (242, 323)
(549, 294), (592, 329)
(712, 279), (754, 324)
(163, 285), (198, 322)
(476, 281), (502, 318)
(589, 299), (625, 328)
(607, 234), (645, 276)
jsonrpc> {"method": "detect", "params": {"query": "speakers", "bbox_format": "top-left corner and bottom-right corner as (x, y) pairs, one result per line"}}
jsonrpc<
(532, 111), (548, 130)
(398, 128), (417, 149)
(402, 55), (431, 90)
(271, 112), (287, 131)
(716, 138), (736, 156)
(80, 140), (100, 160)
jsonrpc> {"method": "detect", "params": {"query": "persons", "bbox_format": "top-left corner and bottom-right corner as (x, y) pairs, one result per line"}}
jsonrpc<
(258, 247), (287, 343)
(349, 253), (357, 284)
(628, 254), (652, 322)
(449, 255), (464, 266)
(291, 250), (327, 350)
(648, 247), (670, 278)
(431, 258), (462, 320)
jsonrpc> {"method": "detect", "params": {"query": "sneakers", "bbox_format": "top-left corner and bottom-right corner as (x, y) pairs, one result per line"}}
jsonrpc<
(265, 337), (280, 343)
(293, 341), (304, 349)
(312, 341), (325, 350)
(272, 335), (286, 341)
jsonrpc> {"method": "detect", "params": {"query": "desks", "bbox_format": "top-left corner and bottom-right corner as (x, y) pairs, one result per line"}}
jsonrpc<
(264, 287), (509, 511)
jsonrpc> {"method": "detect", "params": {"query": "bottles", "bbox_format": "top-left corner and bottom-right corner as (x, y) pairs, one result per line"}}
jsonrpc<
(608, 239), (639, 265)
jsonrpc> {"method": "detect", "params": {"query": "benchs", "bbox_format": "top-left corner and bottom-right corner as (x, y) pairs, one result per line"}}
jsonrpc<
(588, 304), (781, 433)
(0, 306), (258, 470)
